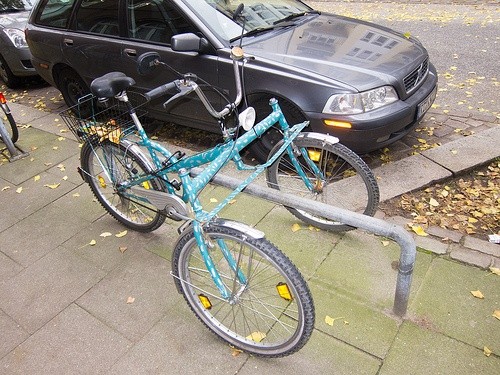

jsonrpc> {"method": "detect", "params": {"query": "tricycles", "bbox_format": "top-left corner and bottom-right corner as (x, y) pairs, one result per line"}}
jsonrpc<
(55, 3), (380, 358)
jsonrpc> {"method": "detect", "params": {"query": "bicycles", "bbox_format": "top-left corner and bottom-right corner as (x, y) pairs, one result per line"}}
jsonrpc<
(0, 91), (18, 143)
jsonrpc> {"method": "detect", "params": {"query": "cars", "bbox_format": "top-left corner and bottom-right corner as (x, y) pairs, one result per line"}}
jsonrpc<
(0, 0), (40, 88)
(26, 0), (438, 171)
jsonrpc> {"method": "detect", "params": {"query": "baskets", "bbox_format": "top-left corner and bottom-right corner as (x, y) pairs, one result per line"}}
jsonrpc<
(60, 89), (149, 146)
